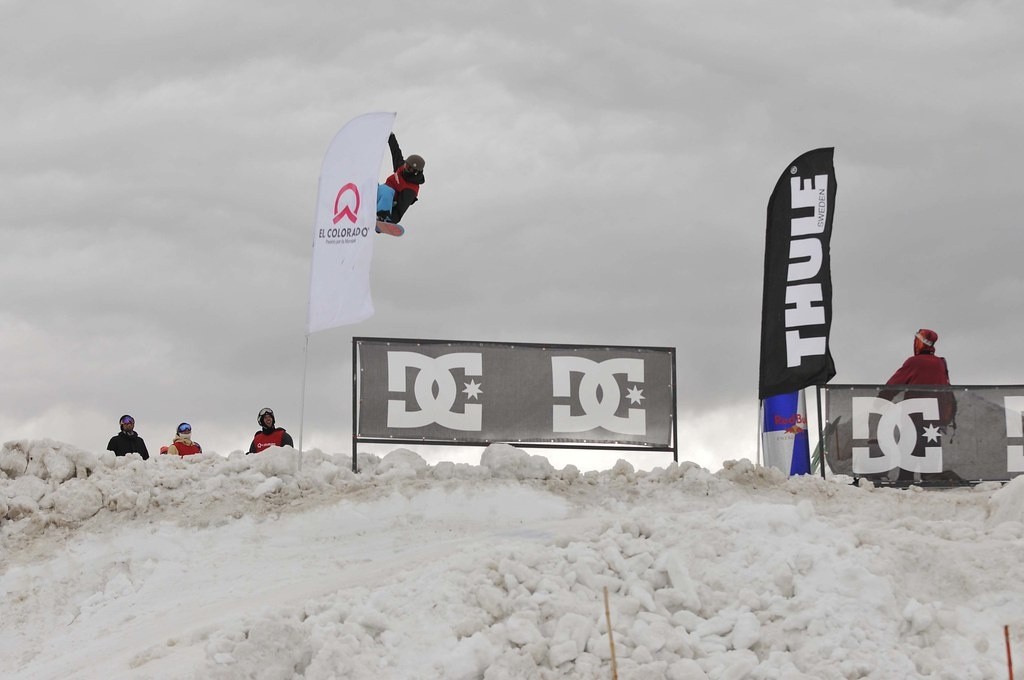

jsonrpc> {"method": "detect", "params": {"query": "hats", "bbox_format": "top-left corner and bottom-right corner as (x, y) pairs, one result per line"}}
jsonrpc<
(405, 155), (425, 171)
(176, 421), (192, 440)
(914, 329), (938, 354)
(119, 415), (135, 431)
(257, 408), (275, 429)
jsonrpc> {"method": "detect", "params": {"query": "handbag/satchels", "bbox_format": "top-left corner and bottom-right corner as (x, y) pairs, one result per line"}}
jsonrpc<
(939, 356), (957, 444)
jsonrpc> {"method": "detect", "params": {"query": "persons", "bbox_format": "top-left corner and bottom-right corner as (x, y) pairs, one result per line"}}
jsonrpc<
(876, 329), (957, 489)
(376, 129), (425, 237)
(160, 422), (203, 457)
(246, 407), (293, 455)
(106, 415), (150, 461)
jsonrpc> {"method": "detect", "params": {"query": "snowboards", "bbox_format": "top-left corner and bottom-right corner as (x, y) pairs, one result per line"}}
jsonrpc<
(376, 220), (405, 237)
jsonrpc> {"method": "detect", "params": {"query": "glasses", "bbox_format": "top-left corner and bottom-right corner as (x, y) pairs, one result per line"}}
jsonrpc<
(258, 408), (273, 418)
(178, 423), (191, 432)
(123, 418), (133, 424)
